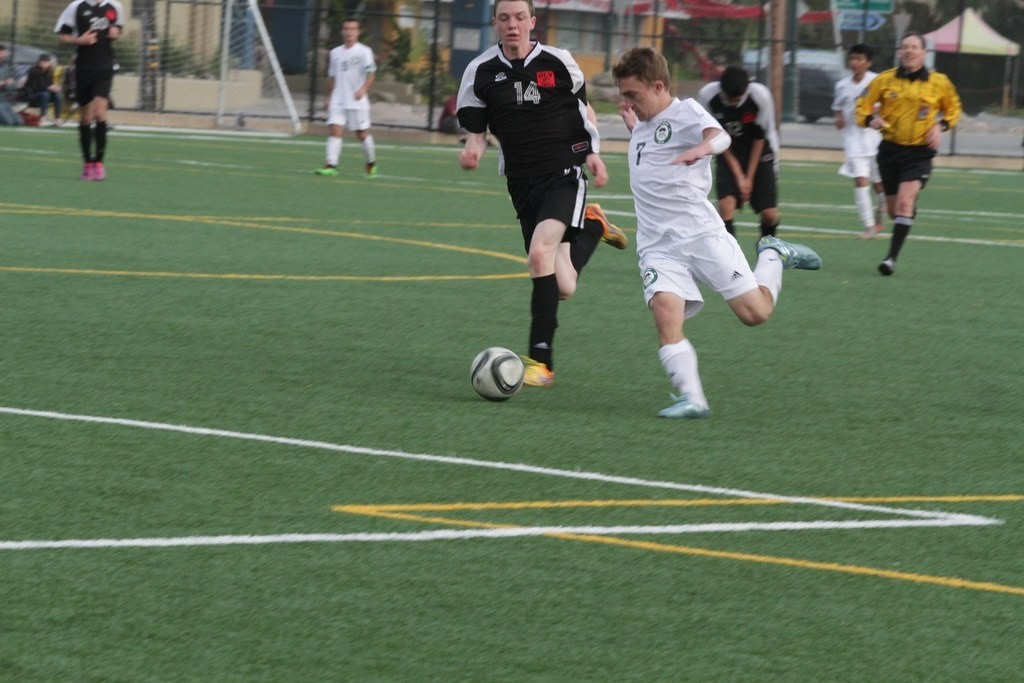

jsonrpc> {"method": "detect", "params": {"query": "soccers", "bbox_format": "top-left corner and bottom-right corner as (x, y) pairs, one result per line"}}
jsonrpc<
(468, 346), (525, 402)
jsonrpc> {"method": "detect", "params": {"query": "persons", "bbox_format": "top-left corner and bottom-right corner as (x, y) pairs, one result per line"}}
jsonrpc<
(455, 0), (628, 386)
(830, 44), (887, 240)
(0, 44), (20, 126)
(439, 96), (459, 133)
(698, 64), (780, 238)
(313, 17), (378, 179)
(54, 0), (124, 181)
(60, 51), (79, 110)
(616, 48), (822, 419)
(20, 53), (63, 126)
(853, 31), (962, 276)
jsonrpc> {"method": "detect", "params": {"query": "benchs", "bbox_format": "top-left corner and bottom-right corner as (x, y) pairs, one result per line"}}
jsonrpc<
(8, 62), (77, 126)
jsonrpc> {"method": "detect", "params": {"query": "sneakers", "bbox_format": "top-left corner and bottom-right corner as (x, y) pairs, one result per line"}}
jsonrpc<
(367, 159), (379, 175)
(757, 235), (822, 270)
(316, 163), (340, 176)
(519, 355), (554, 385)
(656, 393), (710, 418)
(94, 161), (105, 180)
(82, 162), (96, 180)
(585, 203), (627, 249)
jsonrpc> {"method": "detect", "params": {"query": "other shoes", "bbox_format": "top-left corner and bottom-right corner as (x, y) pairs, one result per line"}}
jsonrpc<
(40, 119), (54, 126)
(859, 229), (876, 239)
(879, 257), (895, 275)
(874, 222), (884, 233)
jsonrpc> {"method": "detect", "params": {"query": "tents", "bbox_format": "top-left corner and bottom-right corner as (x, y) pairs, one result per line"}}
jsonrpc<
(923, 7), (1020, 55)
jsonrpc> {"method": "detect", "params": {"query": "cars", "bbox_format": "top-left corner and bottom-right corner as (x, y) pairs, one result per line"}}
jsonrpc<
(0, 41), (58, 79)
(741, 48), (847, 124)
